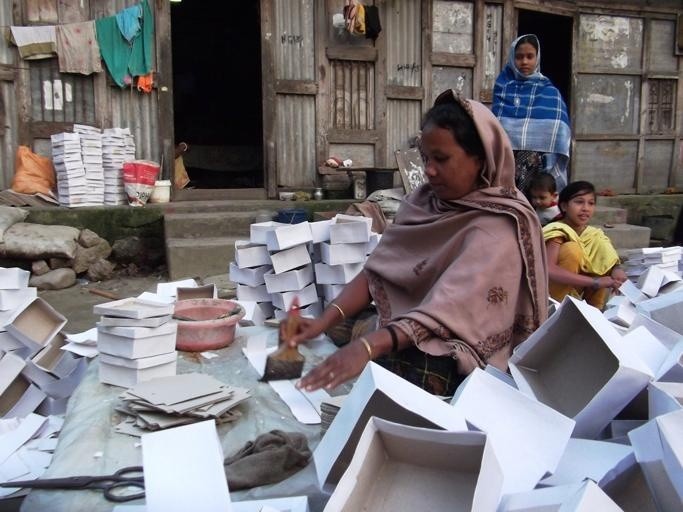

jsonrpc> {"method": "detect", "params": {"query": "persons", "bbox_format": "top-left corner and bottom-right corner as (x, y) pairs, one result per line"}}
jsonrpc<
(530, 171), (561, 227)
(490, 29), (573, 198)
(277, 87), (548, 398)
(544, 179), (628, 312)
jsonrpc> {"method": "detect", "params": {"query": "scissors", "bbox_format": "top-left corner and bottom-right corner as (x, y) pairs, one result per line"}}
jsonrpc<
(1, 467), (145, 502)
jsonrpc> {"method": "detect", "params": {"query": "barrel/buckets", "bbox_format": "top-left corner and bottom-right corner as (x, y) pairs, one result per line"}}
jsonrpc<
(149, 180), (171, 204)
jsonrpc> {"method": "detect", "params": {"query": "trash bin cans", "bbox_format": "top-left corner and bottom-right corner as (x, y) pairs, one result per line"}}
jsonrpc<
(278, 209), (306, 224)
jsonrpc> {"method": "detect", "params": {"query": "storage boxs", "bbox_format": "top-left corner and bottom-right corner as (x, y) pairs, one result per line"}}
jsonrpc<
(1, 267), (30, 292)
(155, 278), (200, 297)
(51, 121), (138, 207)
(139, 419), (233, 510)
(229, 216), (682, 512)
(175, 283), (218, 301)
(1, 390), (73, 505)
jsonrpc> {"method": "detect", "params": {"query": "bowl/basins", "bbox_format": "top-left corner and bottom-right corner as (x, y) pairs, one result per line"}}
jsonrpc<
(169, 298), (246, 352)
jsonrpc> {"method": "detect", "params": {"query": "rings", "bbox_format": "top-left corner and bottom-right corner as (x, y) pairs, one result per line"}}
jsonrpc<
(328, 371), (336, 378)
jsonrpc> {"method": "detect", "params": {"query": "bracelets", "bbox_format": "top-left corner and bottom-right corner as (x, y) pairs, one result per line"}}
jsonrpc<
(360, 338), (375, 361)
(329, 303), (348, 321)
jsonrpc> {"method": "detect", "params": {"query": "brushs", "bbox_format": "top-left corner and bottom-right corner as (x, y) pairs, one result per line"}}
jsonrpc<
(259, 296), (305, 383)
